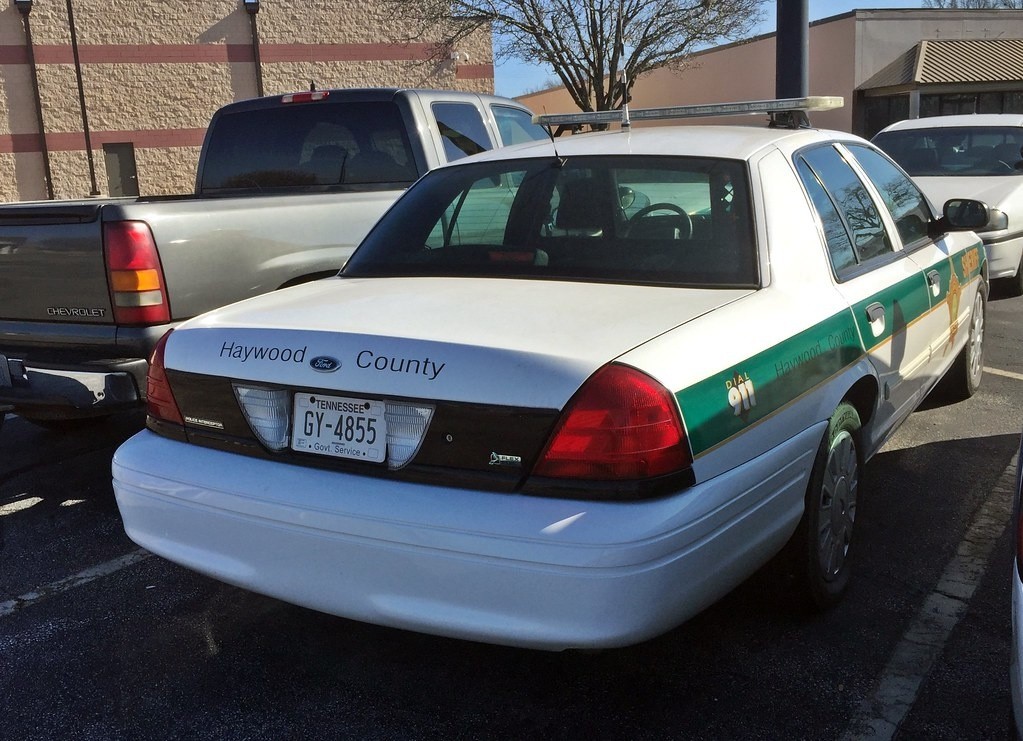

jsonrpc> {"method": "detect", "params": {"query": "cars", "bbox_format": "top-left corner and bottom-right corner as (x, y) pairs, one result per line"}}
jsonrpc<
(869, 113), (1023, 293)
(112, 68), (995, 665)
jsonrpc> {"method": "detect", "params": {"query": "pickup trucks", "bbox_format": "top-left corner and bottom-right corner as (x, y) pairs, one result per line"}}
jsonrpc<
(1, 81), (654, 472)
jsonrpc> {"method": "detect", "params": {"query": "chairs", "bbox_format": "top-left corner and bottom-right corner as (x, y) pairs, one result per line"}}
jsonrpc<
(550, 179), (622, 241)
